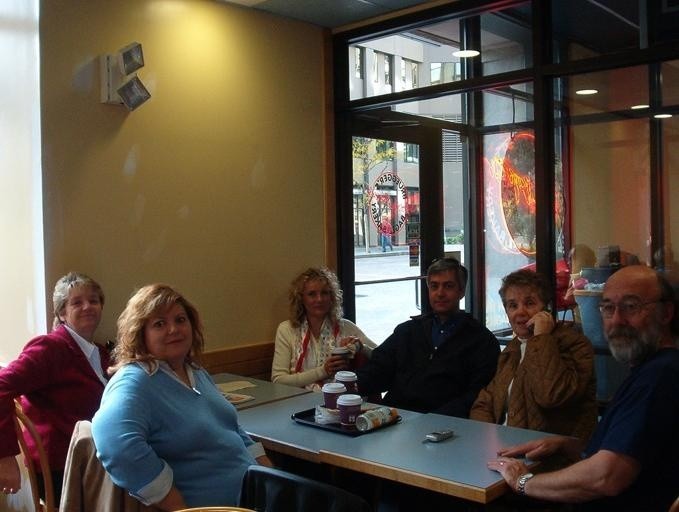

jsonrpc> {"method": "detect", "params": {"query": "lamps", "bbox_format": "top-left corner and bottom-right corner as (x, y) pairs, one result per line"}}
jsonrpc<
(454, 17), (482, 59)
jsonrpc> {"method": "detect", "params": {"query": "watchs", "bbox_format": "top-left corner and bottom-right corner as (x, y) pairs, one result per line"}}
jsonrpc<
(517, 473), (534, 494)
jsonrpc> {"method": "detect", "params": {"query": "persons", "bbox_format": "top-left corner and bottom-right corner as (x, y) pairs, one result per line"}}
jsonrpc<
(380, 217), (393, 251)
(488, 265), (679, 512)
(92, 285), (275, 512)
(468, 270), (595, 434)
(0, 273), (117, 512)
(566, 244), (595, 304)
(271, 268), (378, 385)
(338, 258), (501, 419)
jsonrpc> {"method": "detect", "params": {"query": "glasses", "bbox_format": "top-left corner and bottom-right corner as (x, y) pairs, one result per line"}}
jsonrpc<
(596, 299), (658, 314)
(431, 256), (459, 265)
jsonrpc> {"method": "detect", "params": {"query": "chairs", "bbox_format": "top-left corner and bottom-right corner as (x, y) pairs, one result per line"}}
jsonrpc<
(70, 420), (151, 511)
(241, 463), (371, 510)
(3, 400), (60, 512)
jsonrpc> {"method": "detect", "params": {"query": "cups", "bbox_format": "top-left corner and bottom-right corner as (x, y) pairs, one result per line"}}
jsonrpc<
(323, 382), (347, 408)
(335, 369), (357, 391)
(337, 393), (360, 430)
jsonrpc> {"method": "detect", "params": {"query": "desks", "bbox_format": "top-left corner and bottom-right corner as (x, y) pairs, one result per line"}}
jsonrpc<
(227, 390), (565, 512)
(181, 371), (303, 412)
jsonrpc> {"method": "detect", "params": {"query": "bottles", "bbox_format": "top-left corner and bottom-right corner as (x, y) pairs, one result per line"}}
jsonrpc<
(355, 406), (397, 431)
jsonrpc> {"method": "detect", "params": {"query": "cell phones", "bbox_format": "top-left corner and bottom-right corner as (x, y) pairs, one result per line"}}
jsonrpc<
(422, 427), (453, 445)
(529, 300), (554, 334)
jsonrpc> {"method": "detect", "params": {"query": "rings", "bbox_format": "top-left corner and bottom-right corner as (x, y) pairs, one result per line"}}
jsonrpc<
(500, 461), (506, 465)
